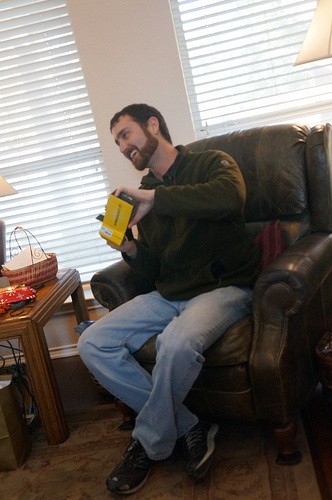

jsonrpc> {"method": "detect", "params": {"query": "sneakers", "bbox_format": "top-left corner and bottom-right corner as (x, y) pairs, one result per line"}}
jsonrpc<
(105, 437), (152, 495)
(182, 421), (217, 478)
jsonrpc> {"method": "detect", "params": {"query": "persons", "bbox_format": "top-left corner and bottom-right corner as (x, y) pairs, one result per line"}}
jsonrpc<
(77, 104), (257, 495)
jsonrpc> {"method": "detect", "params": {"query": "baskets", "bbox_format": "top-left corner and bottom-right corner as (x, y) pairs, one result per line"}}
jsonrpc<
(1, 227), (57, 288)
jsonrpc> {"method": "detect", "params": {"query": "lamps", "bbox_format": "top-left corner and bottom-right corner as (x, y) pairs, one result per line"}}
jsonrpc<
(0, 175), (19, 272)
(290, 0), (332, 72)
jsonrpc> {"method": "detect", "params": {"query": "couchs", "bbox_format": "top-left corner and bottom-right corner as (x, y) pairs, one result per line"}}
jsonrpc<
(90, 123), (332, 464)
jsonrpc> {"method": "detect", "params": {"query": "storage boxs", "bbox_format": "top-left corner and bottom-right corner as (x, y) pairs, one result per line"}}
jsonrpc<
(96, 191), (135, 247)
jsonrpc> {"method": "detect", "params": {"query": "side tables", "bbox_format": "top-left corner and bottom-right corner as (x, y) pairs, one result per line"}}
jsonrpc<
(0, 267), (110, 444)
